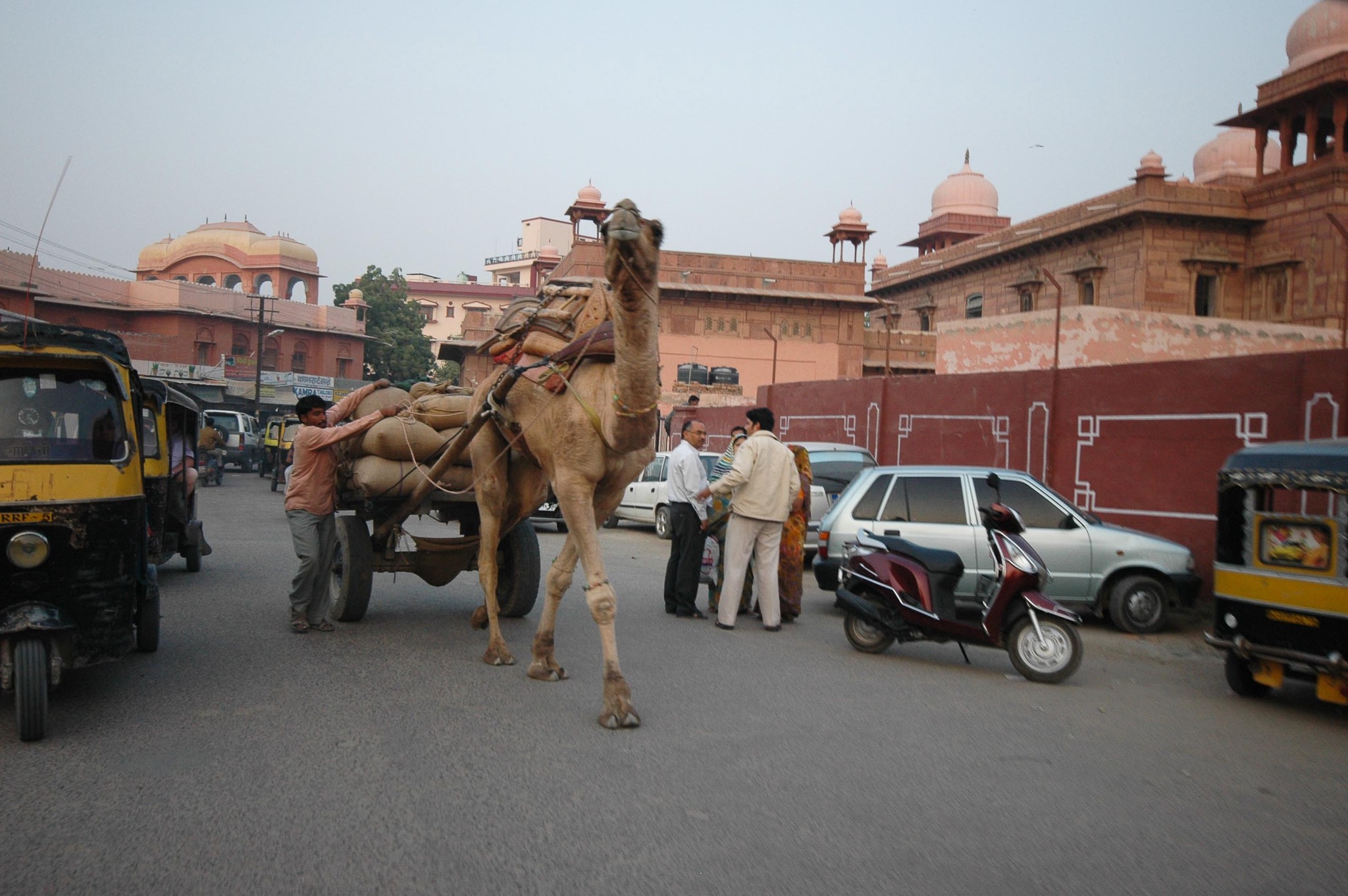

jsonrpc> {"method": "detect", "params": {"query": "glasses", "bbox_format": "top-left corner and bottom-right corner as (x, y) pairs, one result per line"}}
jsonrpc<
(685, 429), (707, 437)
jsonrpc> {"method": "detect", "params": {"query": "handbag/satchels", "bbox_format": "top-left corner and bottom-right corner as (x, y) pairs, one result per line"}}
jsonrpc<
(698, 536), (720, 586)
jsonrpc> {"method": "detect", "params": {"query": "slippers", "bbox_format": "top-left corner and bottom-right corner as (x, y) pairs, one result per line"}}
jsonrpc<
(290, 607), (311, 632)
(311, 619), (334, 631)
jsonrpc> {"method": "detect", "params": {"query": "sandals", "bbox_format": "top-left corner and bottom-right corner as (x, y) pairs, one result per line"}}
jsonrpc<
(677, 609), (708, 620)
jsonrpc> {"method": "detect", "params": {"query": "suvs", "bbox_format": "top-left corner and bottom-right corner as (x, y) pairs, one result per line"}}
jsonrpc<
(200, 408), (265, 474)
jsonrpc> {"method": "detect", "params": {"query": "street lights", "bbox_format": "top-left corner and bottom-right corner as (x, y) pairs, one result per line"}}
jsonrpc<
(254, 329), (286, 417)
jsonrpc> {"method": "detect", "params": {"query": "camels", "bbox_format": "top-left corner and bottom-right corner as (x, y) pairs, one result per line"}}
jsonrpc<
(463, 198), (666, 730)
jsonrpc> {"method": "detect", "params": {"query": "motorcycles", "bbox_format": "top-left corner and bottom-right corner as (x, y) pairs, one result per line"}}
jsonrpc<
(46, 375), (213, 574)
(258, 413), (302, 492)
(1203, 439), (1348, 708)
(0, 309), (165, 742)
(196, 443), (225, 487)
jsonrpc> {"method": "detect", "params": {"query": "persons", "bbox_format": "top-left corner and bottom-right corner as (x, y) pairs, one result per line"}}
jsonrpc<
(199, 418), (228, 469)
(168, 415), (199, 498)
(284, 379), (401, 633)
(700, 407), (813, 633)
(664, 395), (700, 437)
(663, 420), (712, 619)
(284, 442), (294, 493)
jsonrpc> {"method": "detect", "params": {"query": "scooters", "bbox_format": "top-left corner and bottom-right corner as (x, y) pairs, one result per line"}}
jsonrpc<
(832, 471), (1087, 685)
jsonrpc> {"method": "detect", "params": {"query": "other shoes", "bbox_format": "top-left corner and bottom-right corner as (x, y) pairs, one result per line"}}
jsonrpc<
(764, 622), (782, 631)
(715, 619), (733, 629)
(217, 470), (223, 476)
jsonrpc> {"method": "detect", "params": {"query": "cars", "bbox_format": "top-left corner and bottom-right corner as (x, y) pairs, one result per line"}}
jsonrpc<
(781, 440), (882, 568)
(528, 450), (723, 539)
(811, 464), (1204, 636)
